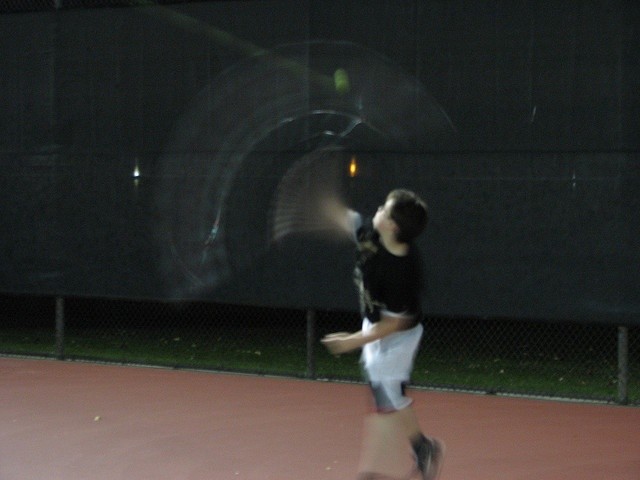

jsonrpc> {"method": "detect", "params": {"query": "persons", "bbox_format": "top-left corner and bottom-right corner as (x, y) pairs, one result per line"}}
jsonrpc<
(266, 144), (459, 479)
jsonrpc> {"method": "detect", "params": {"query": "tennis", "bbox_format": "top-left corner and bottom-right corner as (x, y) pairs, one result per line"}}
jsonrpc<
(334, 69), (349, 93)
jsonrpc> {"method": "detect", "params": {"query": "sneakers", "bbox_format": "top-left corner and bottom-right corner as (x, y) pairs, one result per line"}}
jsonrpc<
(417, 437), (443, 480)
